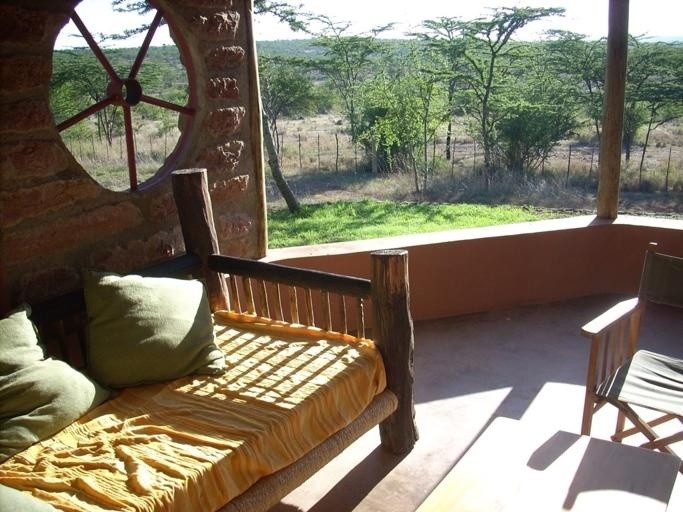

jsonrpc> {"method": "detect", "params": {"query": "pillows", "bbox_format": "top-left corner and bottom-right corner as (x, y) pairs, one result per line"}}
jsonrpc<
(0, 303), (111, 465)
(82, 267), (225, 389)
(1, 483), (57, 512)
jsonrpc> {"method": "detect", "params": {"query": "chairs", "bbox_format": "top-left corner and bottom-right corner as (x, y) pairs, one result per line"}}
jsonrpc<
(581, 242), (683, 474)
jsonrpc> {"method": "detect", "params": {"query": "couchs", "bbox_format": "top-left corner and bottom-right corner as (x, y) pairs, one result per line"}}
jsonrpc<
(0, 168), (420, 512)
